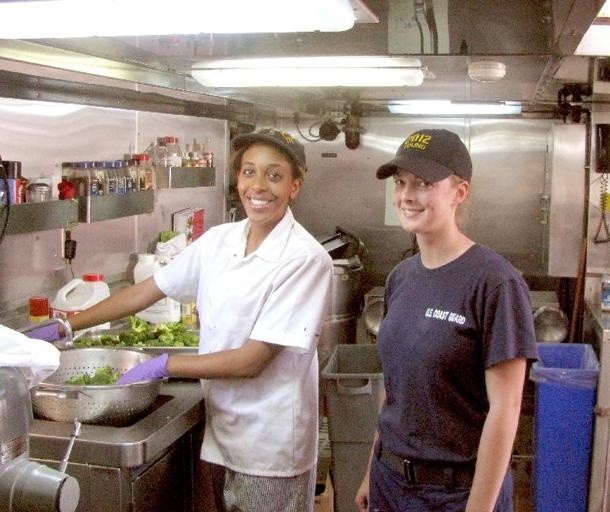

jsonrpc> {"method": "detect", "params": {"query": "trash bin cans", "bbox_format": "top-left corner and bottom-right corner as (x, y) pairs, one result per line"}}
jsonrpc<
(529, 342), (600, 512)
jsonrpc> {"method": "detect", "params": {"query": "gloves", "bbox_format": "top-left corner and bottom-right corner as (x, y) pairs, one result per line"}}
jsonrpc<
(114, 351), (170, 385)
(23, 322), (62, 343)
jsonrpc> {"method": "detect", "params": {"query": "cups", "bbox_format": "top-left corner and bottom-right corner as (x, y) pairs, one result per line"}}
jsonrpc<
(601, 271), (610, 314)
(29, 296), (50, 322)
(27, 184), (51, 203)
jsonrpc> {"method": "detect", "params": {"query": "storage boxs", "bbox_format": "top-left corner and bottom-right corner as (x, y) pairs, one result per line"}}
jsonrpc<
(322, 344), (386, 443)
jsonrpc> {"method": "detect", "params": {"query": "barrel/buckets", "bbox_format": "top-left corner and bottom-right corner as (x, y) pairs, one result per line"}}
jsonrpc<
(316, 266), (362, 381)
(133, 254), (182, 325)
(51, 274), (111, 332)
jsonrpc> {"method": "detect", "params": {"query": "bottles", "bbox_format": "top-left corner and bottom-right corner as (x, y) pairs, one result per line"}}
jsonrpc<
(152, 136), (215, 168)
(0, 162), (24, 205)
(181, 303), (196, 326)
(63, 153), (152, 194)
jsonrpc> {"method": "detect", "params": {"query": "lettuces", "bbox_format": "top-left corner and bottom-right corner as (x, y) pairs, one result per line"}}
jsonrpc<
(74, 315), (199, 347)
(65, 365), (120, 386)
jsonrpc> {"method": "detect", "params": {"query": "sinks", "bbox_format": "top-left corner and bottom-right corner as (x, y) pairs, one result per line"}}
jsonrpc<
(31, 393), (174, 428)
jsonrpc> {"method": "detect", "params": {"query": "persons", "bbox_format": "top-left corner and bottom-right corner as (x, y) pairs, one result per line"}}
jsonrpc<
(19, 129), (334, 512)
(354, 129), (539, 512)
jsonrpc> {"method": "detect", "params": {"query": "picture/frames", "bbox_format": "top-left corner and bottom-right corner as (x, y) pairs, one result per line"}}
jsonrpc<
(588, 56), (610, 100)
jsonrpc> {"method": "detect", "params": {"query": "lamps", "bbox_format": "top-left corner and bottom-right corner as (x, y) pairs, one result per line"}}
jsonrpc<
(190, 55), (424, 88)
(0, 1), (355, 39)
(468, 60), (506, 83)
(388, 100), (522, 114)
(293, 111), (341, 143)
(341, 112), (366, 149)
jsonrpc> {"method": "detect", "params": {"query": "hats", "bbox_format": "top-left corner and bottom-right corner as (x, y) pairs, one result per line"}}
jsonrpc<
(377, 130), (472, 184)
(232, 127), (306, 169)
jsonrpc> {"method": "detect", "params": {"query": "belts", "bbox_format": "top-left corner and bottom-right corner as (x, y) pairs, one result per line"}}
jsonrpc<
(379, 449), (454, 484)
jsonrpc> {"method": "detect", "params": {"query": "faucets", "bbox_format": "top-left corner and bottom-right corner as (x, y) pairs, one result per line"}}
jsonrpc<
(17, 316), (74, 346)
